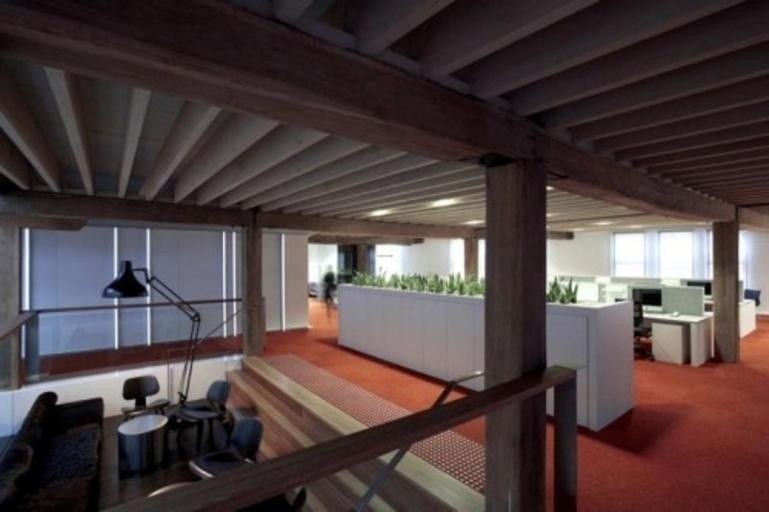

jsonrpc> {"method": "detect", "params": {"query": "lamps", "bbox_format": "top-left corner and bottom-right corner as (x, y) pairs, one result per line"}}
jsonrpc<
(101, 259), (201, 430)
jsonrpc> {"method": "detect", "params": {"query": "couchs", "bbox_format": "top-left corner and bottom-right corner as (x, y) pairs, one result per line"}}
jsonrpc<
(0, 386), (109, 512)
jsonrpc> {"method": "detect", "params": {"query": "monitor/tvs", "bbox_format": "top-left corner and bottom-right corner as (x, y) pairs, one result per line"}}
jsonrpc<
(686, 281), (712, 298)
(632, 288), (663, 312)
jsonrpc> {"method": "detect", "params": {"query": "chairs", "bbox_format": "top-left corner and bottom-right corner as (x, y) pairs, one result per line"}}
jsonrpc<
(172, 372), (233, 450)
(744, 289), (761, 307)
(613, 298), (655, 362)
(120, 373), (169, 425)
(188, 415), (262, 481)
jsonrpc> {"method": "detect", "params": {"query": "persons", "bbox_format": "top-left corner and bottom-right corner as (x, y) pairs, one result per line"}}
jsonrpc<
(322, 265), (337, 303)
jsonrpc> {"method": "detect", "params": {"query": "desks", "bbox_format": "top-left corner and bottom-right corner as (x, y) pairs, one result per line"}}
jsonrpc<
(632, 300), (716, 366)
(705, 295), (757, 340)
